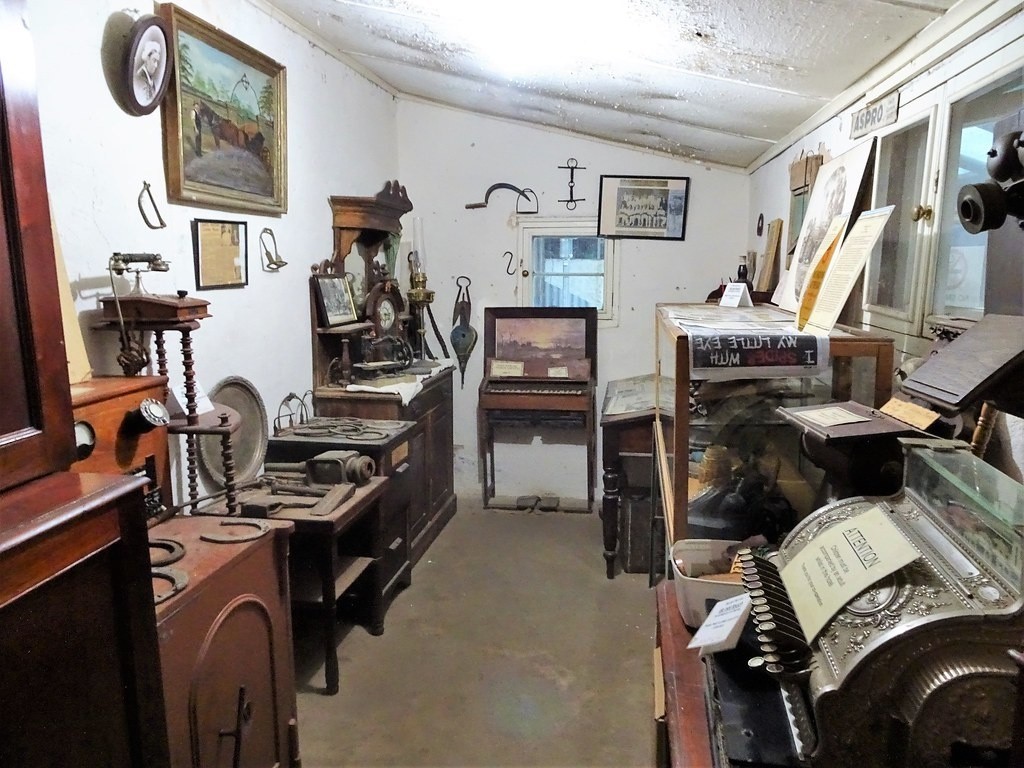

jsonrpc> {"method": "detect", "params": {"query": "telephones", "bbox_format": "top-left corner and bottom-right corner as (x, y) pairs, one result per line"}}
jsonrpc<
(98, 252), (212, 322)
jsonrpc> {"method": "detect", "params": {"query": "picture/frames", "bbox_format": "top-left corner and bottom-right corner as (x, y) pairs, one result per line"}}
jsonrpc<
(313, 273), (358, 328)
(123, 14), (174, 117)
(161, 4), (288, 214)
(484, 306), (597, 386)
(597, 174), (690, 241)
(1, 0), (79, 491)
(195, 218), (248, 290)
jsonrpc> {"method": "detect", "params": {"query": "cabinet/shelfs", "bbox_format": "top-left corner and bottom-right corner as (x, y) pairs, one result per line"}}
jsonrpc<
(266, 417), (419, 637)
(309, 180), (458, 570)
(0, 472), (172, 767)
(148, 515), (302, 768)
(649, 303), (895, 591)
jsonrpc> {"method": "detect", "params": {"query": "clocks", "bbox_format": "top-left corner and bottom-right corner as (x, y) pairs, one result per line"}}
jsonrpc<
(366, 282), (406, 376)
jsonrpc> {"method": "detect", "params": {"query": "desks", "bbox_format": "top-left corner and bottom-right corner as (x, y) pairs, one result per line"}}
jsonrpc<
(655, 580), (715, 768)
(599, 374), (656, 579)
(197, 477), (391, 695)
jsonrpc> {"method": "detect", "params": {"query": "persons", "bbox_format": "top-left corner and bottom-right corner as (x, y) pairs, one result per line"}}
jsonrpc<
(615, 190), (684, 234)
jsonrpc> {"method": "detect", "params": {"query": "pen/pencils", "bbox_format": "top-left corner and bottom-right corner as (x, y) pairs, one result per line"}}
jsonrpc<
(720, 276), (738, 294)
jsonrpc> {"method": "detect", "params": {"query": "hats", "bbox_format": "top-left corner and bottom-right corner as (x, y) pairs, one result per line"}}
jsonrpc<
(141, 41), (161, 61)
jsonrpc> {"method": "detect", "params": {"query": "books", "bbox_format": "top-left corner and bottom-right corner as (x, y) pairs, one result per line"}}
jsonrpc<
(757, 218), (783, 293)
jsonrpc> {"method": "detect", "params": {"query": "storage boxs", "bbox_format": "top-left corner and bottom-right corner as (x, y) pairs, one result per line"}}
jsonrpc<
(669, 539), (746, 627)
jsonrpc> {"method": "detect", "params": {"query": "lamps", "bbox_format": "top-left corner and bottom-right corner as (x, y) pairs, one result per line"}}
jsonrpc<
(406, 216), (442, 368)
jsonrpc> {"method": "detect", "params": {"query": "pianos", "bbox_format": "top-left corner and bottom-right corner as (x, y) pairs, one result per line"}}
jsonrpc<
(472, 352), (600, 516)
